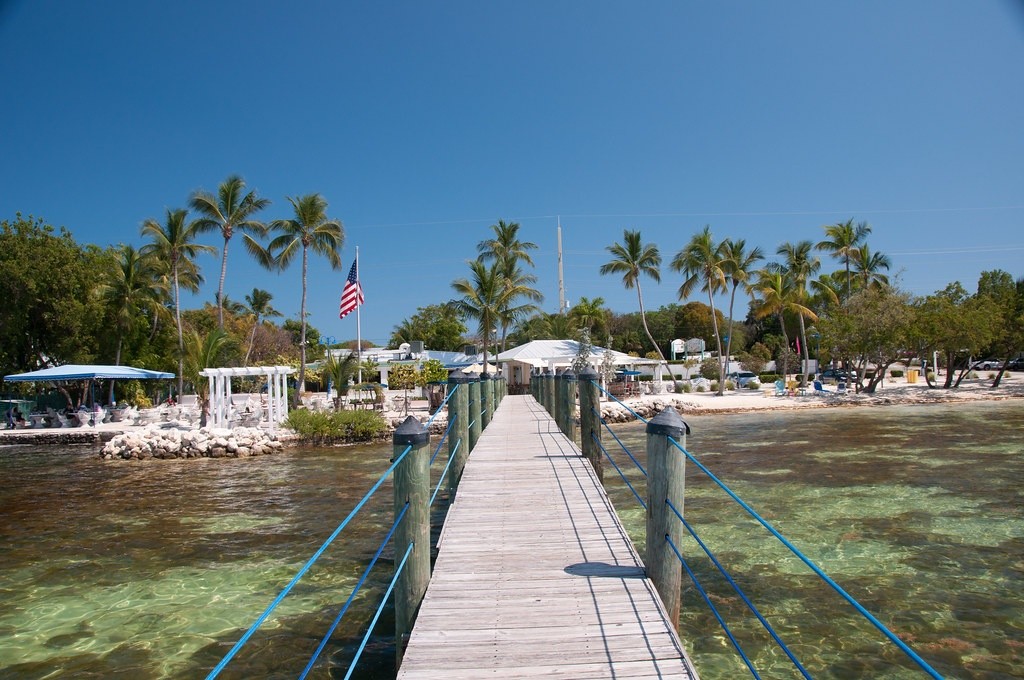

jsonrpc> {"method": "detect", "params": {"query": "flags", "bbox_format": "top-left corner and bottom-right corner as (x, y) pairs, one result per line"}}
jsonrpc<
(339, 259), (365, 320)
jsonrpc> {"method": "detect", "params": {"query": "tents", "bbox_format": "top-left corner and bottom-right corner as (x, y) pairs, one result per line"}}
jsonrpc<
(4, 364), (175, 428)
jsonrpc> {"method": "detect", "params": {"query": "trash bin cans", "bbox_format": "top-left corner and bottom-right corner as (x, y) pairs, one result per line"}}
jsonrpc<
(907, 370), (918, 383)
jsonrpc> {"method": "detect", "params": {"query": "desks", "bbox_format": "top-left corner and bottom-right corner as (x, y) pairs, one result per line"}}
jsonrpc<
(314, 398), (327, 410)
(29, 414), (48, 427)
(174, 407), (190, 419)
(110, 409), (122, 422)
(262, 404), (275, 407)
(262, 407), (276, 421)
(68, 412), (94, 427)
(179, 404), (195, 406)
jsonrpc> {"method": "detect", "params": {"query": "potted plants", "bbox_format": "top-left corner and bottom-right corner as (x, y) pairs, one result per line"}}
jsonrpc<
(392, 395), (412, 411)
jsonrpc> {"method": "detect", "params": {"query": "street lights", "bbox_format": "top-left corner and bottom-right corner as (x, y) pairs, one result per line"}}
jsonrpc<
(320, 334), (336, 394)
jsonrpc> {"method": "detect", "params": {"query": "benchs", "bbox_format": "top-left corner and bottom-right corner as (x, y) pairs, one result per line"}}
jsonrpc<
(374, 400), (390, 412)
(342, 399), (353, 410)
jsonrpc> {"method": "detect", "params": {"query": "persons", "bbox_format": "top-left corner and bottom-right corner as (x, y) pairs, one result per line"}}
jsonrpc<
(196, 397), (210, 430)
(37, 382), (89, 413)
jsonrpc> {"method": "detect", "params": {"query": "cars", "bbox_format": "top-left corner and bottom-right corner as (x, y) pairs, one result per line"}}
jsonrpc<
(972, 358), (1004, 371)
(819, 368), (859, 385)
(1008, 357), (1024, 371)
(730, 370), (761, 389)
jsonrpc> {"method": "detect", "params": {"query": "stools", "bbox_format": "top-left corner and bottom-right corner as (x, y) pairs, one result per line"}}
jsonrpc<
(799, 389), (806, 397)
(763, 390), (771, 399)
(788, 391), (795, 396)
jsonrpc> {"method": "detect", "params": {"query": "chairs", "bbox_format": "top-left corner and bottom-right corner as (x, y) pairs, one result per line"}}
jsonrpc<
(774, 380), (786, 397)
(814, 382), (830, 395)
(10, 404), (140, 428)
(787, 380), (798, 396)
(837, 382), (847, 396)
